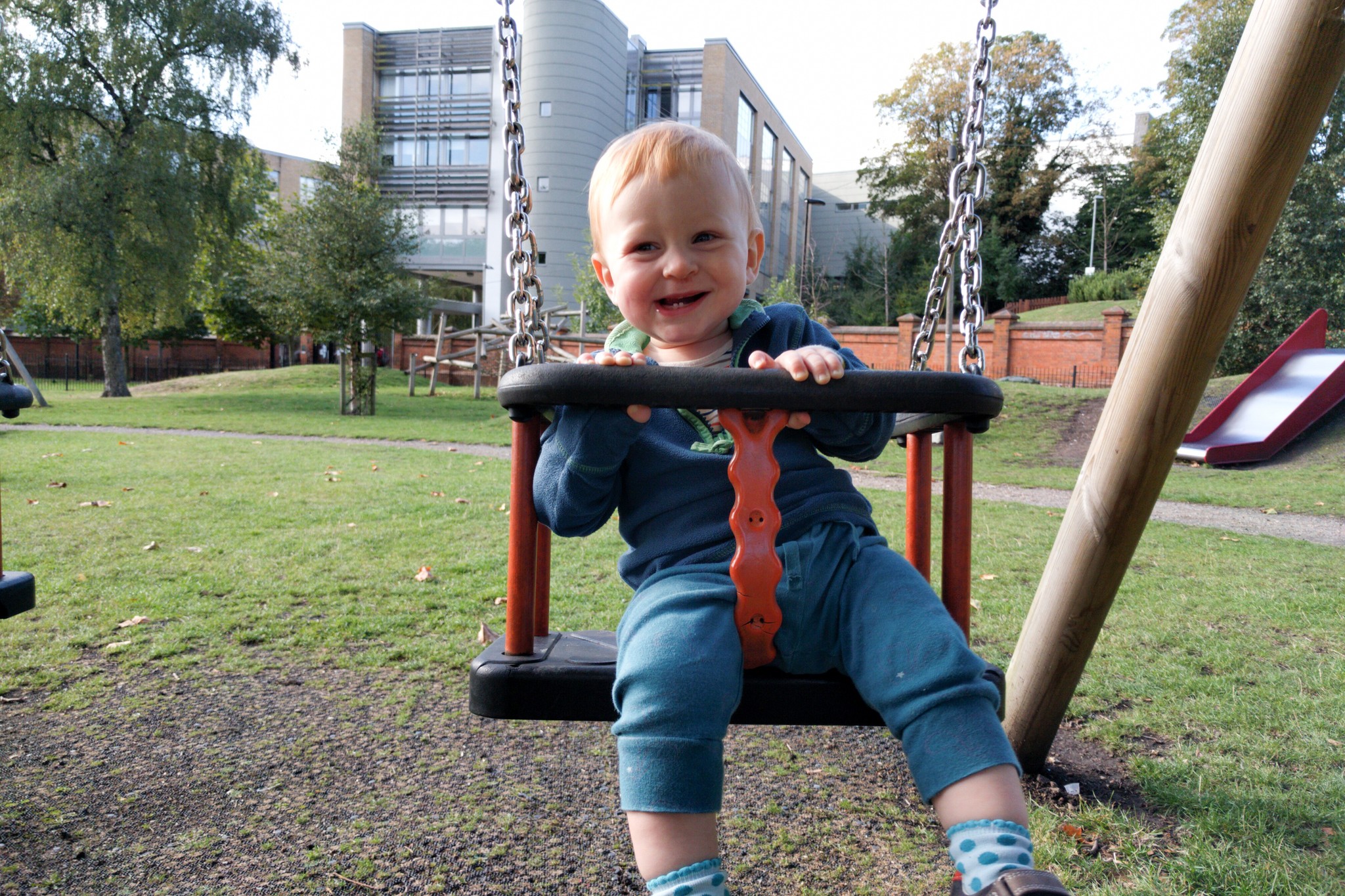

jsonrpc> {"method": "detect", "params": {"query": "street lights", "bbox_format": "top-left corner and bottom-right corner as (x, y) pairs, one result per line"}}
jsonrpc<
(1084, 194), (1105, 273)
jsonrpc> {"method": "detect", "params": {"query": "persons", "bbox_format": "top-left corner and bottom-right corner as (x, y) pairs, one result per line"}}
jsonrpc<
(376, 345), (388, 367)
(532, 121), (1073, 896)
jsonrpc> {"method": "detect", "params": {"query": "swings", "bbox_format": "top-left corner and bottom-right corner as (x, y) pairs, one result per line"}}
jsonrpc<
(470, 0), (1005, 725)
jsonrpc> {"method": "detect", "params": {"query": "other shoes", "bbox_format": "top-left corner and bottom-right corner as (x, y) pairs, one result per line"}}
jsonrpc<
(951, 871), (1069, 896)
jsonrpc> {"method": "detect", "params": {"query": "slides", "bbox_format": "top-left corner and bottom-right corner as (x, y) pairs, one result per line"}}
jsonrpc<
(1173, 307), (1345, 466)
